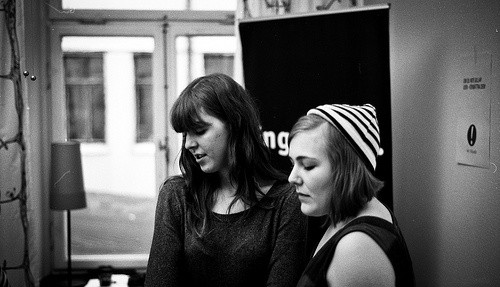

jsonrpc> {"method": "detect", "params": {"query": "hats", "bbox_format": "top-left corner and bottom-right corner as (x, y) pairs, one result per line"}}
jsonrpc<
(307, 103), (382, 171)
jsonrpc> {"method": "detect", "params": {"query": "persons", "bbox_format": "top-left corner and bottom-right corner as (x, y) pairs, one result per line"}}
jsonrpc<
(287, 102), (415, 287)
(143, 72), (331, 287)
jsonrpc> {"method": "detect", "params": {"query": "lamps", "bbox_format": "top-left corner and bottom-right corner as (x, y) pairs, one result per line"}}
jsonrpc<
(39, 139), (87, 286)
